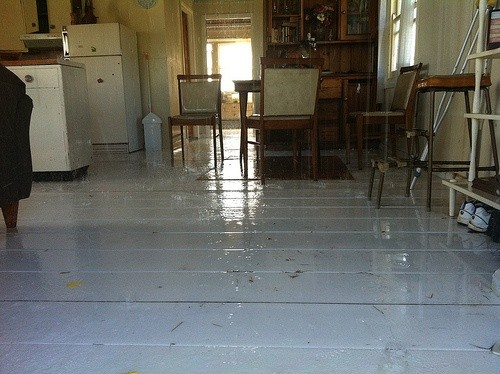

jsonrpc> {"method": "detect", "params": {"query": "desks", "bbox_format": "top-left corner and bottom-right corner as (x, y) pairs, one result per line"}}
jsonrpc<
(234, 79), (349, 177)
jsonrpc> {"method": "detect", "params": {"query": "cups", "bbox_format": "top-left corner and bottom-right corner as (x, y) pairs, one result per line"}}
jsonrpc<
(273, 0), (289, 15)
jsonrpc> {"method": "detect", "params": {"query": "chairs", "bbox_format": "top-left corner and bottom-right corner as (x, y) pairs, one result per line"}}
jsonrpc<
(348, 64), (423, 170)
(167, 73), (223, 168)
(257, 56), (326, 182)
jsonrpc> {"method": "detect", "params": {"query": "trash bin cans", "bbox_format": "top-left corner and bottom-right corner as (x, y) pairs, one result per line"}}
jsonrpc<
(142, 111), (162, 149)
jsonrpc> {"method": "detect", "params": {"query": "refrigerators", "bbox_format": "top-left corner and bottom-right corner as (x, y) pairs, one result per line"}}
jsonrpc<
(61, 22), (145, 154)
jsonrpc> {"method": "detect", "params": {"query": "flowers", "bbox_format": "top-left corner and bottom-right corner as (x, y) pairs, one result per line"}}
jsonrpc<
(305, 5), (335, 25)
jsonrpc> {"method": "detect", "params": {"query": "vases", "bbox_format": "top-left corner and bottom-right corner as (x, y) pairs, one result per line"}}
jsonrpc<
(311, 22), (329, 40)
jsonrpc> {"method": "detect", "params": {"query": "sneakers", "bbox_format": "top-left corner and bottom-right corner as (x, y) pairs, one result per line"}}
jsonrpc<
(468, 207), (498, 231)
(457, 200), (487, 226)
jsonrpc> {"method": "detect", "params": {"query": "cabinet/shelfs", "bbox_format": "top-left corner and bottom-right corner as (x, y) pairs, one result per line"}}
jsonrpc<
(265, 0), (304, 45)
(441, 0), (500, 215)
(337, 0), (380, 41)
(222, 103), (253, 120)
(21, 65), (65, 171)
(22, 0), (73, 34)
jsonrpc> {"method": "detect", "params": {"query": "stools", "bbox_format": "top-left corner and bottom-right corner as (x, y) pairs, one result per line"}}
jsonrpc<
(405, 73), (498, 211)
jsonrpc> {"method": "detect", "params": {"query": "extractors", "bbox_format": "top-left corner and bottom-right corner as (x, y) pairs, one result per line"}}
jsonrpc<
(19, 33), (63, 48)
